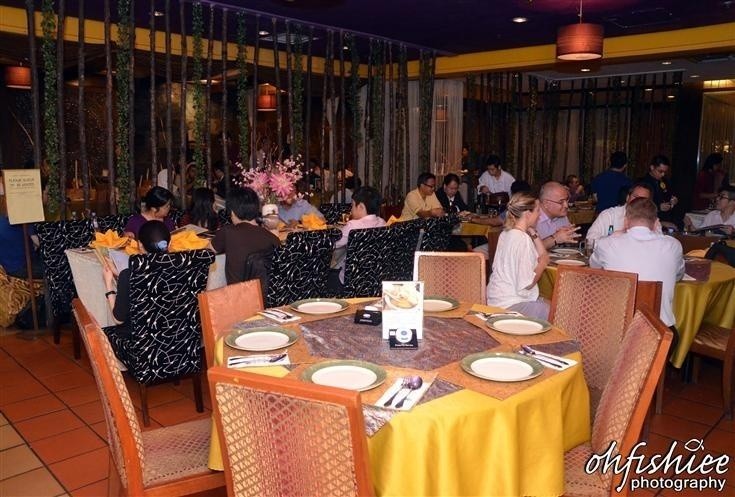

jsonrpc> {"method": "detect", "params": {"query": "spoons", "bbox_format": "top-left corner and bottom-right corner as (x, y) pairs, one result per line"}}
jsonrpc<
(515, 344), (567, 370)
(396, 376), (424, 407)
(228, 350), (287, 366)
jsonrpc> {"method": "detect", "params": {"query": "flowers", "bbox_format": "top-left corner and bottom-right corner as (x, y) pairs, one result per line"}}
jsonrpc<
(231, 148), (314, 205)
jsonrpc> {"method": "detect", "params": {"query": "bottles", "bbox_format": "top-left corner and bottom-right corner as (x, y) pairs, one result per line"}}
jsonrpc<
(454, 206), (496, 222)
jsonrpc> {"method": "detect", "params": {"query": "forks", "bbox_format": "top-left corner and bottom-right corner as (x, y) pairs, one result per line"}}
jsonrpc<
(262, 307), (291, 320)
(384, 376), (411, 407)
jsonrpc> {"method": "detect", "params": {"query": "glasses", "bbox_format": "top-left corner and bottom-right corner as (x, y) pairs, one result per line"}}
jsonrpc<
(717, 193), (729, 200)
(545, 197), (569, 205)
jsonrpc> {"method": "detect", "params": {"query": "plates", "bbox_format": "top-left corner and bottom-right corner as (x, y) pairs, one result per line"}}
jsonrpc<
(490, 315), (553, 334)
(569, 200), (595, 212)
(547, 246), (587, 267)
(385, 291), (419, 312)
(292, 299), (350, 315)
(72, 247), (92, 253)
(464, 351), (545, 380)
(225, 327), (298, 352)
(303, 361), (387, 392)
(423, 293), (459, 311)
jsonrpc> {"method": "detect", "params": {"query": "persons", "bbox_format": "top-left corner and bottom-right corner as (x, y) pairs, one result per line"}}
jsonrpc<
(0, 182), (50, 330)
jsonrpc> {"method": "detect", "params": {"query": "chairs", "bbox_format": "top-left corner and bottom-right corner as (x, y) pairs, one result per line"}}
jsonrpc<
(30, 201), (735, 497)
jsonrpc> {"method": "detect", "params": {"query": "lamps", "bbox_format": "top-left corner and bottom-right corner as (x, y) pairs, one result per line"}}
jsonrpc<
(555, 1), (605, 62)
(4, 60), (32, 89)
(256, 81), (279, 112)
